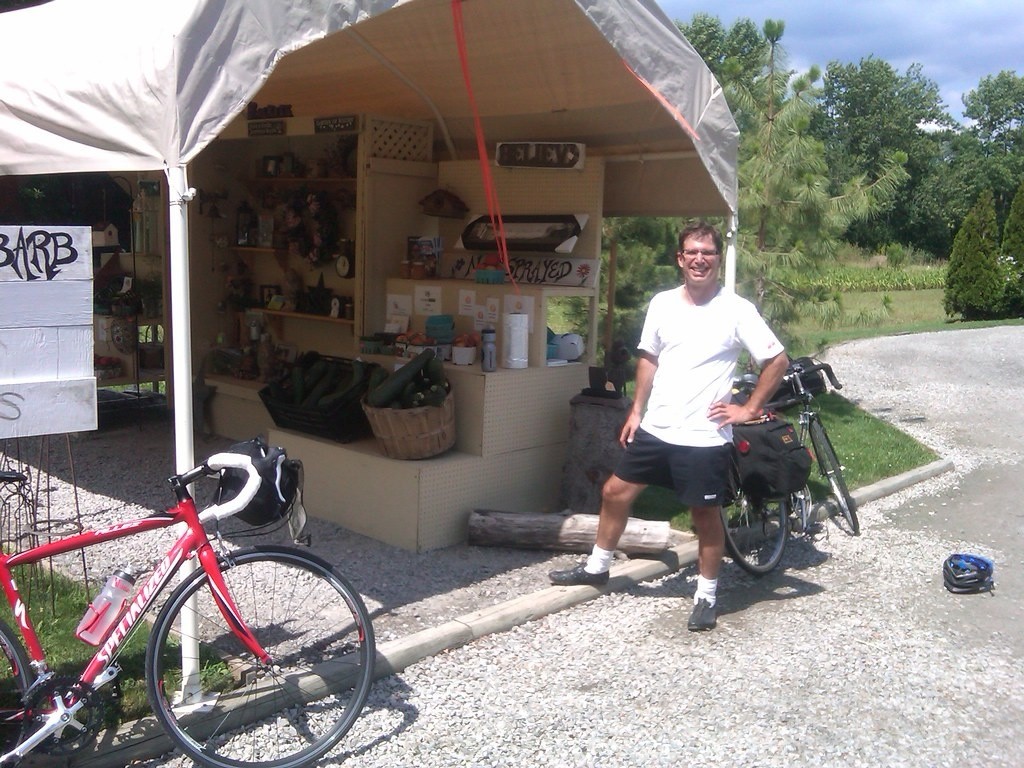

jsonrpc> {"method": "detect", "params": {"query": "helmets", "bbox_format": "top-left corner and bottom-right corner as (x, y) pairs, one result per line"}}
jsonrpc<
(216, 439), (296, 524)
(943, 554), (994, 593)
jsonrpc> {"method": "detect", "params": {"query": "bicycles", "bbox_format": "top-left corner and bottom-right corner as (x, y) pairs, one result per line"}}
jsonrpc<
(720, 356), (859, 575)
(0, 435), (376, 768)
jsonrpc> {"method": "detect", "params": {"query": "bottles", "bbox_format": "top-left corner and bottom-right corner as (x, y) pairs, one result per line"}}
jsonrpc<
(76, 565), (137, 647)
(236, 198), (253, 246)
(481, 322), (497, 372)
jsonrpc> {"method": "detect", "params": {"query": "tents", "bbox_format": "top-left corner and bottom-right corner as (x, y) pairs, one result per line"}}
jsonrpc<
(0, 0), (744, 713)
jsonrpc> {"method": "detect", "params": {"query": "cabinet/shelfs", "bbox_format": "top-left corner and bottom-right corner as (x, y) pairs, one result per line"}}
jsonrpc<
(195, 114), (434, 443)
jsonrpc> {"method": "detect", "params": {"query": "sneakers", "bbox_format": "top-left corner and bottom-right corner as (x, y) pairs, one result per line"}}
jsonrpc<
(688, 599), (717, 629)
(549, 561), (610, 585)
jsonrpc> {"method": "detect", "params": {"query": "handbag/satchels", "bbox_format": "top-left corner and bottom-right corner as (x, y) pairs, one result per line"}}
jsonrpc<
(734, 418), (812, 500)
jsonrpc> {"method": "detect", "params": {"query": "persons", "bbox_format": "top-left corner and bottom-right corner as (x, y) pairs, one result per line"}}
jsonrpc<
(550, 223), (791, 631)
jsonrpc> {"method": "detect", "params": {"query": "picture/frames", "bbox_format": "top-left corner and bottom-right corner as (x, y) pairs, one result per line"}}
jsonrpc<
(260, 284), (282, 308)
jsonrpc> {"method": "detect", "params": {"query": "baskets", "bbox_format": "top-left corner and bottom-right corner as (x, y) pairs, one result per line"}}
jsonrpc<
(362, 385), (457, 460)
(257, 351), (379, 443)
(215, 461), (307, 545)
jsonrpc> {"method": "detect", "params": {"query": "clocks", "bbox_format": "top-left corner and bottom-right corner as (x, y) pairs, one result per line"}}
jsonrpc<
(335, 251), (355, 278)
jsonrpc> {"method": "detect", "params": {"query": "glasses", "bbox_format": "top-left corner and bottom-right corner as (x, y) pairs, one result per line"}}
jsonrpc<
(679, 250), (720, 261)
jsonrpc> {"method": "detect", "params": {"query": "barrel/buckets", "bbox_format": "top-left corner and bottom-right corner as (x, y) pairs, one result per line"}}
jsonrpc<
(361, 376), (456, 460)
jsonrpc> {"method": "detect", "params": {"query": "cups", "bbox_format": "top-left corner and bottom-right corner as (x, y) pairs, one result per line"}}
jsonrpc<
(452, 346), (477, 365)
